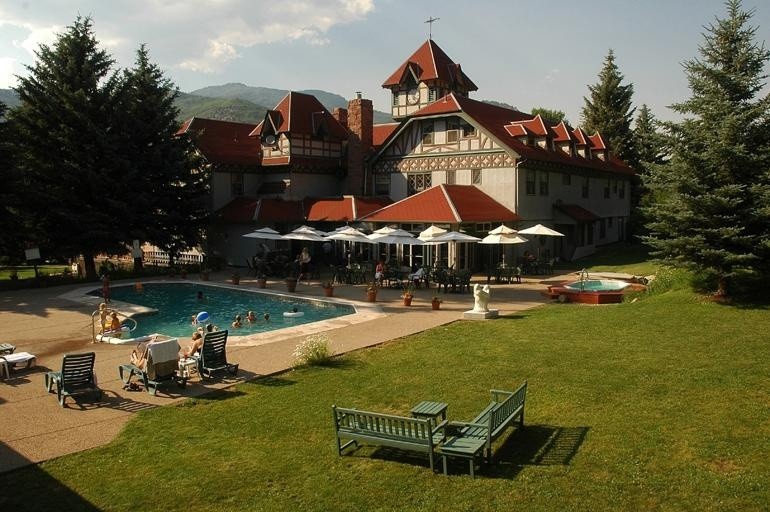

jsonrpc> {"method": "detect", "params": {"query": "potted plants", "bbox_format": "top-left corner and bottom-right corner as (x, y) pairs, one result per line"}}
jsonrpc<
(201, 262), (212, 281)
(286, 268), (298, 292)
(232, 272), (240, 285)
(324, 277), (335, 298)
(366, 279), (377, 302)
(402, 285), (415, 307)
(256, 267), (267, 288)
(180, 267), (187, 279)
(431, 296), (442, 308)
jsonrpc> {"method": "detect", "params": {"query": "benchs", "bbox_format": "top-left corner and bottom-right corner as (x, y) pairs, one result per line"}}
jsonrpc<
(441, 379), (528, 478)
(332, 401), (448, 471)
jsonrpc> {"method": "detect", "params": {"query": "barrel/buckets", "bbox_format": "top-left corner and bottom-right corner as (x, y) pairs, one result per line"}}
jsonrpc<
(202, 269), (212, 281)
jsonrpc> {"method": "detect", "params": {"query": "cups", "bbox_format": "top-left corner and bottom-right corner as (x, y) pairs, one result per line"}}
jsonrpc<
(183, 353), (187, 362)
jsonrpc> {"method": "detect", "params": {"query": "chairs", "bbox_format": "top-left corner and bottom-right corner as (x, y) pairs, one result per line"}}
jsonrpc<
(245, 247), (321, 281)
(331, 255), (400, 288)
(178, 329), (240, 380)
(0, 351), (39, 379)
(406, 262), (472, 294)
(44, 352), (102, 408)
(119, 338), (188, 395)
(486, 263), (521, 284)
(519, 254), (555, 276)
(0, 342), (18, 367)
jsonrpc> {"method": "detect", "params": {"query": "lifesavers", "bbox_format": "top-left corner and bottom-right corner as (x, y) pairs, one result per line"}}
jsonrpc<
(284, 312), (304, 318)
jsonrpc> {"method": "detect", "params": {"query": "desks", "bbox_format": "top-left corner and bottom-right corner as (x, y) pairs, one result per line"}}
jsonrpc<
(410, 398), (449, 427)
(433, 435), (487, 477)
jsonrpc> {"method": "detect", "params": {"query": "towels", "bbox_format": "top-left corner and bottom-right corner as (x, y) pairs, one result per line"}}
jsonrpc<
(146, 339), (179, 364)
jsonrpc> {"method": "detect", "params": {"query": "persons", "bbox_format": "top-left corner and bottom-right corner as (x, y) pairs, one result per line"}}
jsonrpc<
(94, 270), (301, 392)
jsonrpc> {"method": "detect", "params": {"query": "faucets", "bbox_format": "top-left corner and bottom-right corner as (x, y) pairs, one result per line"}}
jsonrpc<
(580, 268), (589, 292)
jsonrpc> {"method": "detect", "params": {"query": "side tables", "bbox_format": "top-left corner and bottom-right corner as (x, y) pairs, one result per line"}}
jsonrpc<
(178, 357), (201, 384)
